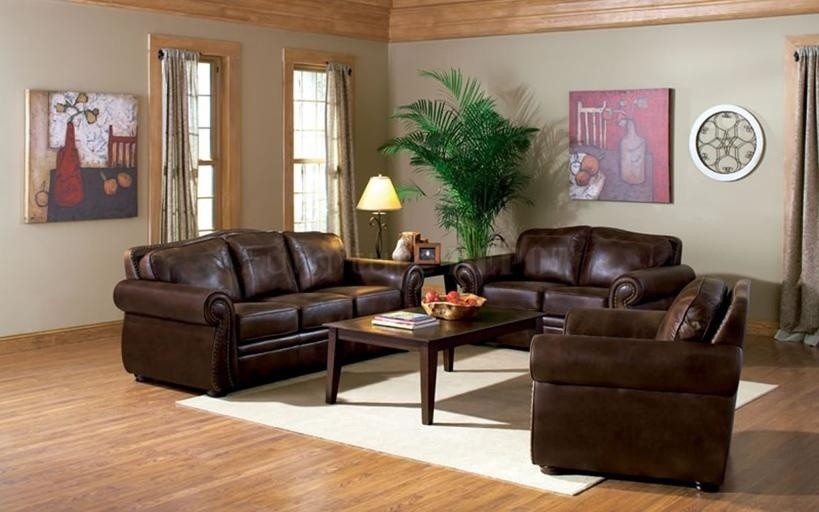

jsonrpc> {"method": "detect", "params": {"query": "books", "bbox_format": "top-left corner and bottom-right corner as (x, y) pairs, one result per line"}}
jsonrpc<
(371, 310), (441, 330)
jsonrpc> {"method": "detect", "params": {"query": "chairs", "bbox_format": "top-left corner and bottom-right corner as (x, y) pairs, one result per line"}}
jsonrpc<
(529, 274), (752, 493)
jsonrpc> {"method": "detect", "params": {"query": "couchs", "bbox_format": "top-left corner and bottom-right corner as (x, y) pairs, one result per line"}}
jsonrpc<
(452, 225), (698, 352)
(113, 229), (425, 398)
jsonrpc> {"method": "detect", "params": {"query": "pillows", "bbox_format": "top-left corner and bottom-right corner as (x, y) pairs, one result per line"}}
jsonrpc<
(655, 277), (728, 342)
(515, 226), (677, 288)
(139, 232), (347, 303)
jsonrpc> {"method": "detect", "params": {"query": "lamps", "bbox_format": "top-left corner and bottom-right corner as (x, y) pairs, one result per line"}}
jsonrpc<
(355, 173), (404, 259)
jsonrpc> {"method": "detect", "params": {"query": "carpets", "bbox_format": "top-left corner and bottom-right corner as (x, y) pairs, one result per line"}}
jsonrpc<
(175, 344), (780, 496)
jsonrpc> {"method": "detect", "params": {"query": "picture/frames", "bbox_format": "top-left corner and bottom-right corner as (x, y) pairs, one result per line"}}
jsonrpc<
(688, 104), (765, 182)
(414, 242), (442, 265)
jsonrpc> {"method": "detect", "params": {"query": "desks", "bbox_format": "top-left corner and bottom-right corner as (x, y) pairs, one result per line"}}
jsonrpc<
(416, 261), (460, 306)
